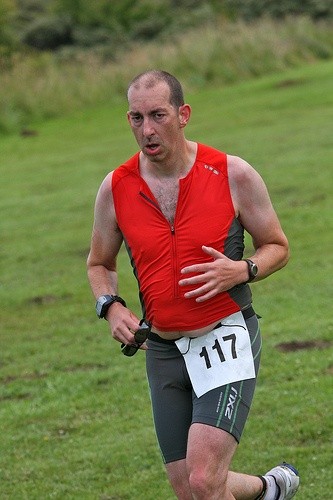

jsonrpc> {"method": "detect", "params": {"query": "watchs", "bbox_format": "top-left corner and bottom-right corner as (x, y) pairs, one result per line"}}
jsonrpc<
(95, 294), (128, 321)
(240, 259), (258, 285)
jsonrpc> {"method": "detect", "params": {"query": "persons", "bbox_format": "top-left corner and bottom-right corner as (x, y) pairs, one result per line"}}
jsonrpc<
(87, 68), (301, 500)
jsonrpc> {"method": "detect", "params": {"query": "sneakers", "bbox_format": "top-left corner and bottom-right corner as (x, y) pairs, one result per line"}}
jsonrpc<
(262, 461), (299, 499)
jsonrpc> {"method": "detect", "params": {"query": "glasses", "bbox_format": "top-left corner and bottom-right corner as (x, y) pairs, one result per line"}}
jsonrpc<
(120, 319), (151, 357)
